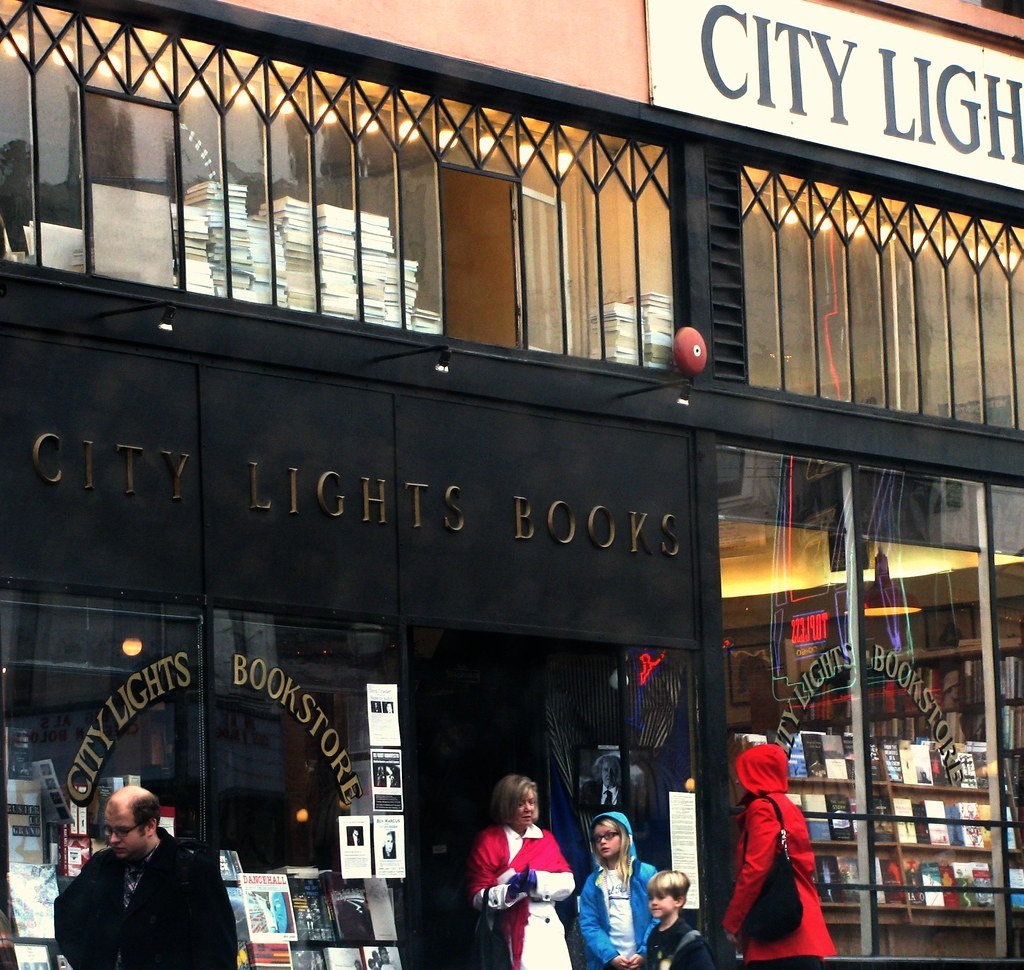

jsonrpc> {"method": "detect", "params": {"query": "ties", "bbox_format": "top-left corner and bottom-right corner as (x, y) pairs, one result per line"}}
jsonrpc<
(604, 790), (612, 805)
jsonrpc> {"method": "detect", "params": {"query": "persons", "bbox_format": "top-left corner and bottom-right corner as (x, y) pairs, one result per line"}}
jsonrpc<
(577, 811), (661, 970)
(53, 785), (238, 970)
(386, 701), (395, 713)
(720, 745), (837, 970)
(582, 754), (627, 806)
(646, 870), (716, 970)
(383, 831), (397, 859)
(368, 946), (398, 969)
(468, 774), (575, 970)
(347, 827), (363, 847)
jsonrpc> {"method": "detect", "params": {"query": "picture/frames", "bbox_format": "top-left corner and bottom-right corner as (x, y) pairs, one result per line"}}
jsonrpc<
(727, 640), (779, 707)
(569, 744), (654, 812)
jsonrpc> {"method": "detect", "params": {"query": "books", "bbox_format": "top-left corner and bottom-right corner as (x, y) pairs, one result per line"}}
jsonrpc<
(734, 724), (1024, 907)
(5, 726), (175, 970)
(218, 847), (398, 942)
(236, 940), (363, 970)
(170, 182), (441, 335)
(360, 946), (402, 970)
(588, 291), (674, 371)
(809, 652), (1023, 750)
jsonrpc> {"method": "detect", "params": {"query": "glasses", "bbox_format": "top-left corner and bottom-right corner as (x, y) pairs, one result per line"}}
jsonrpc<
(593, 832), (619, 842)
(102, 824), (141, 837)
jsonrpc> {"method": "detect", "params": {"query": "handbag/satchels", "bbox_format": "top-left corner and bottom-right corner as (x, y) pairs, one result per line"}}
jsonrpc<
(463, 886), (511, 970)
(742, 796), (803, 946)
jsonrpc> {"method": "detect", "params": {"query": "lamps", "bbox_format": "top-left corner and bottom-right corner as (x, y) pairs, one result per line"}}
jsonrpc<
(372, 343), (451, 374)
(95, 301), (175, 333)
(843, 541), (923, 617)
(613, 380), (692, 405)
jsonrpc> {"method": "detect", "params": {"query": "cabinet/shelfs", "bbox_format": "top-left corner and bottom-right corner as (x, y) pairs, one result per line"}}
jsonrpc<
(726, 646), (1024, 926)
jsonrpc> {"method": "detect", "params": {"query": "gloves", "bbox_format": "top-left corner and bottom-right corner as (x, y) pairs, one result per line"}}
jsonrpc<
(507, 864), (537, 900)
